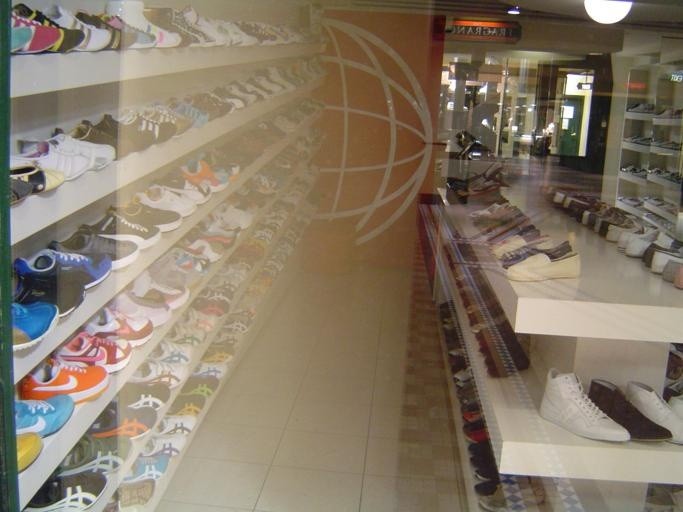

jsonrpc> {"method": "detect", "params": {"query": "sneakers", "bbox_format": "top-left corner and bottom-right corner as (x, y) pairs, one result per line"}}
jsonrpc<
(469, 201), (580, 280)
(539, 367), (683, 444)
(439, 242), (549, 511)
(622, 102), (683, 188)
(545, 186), (683, 287)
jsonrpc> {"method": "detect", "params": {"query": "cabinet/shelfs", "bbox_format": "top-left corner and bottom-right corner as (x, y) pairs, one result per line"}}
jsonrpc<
(616, 65), (681, 242)
(417, 188), (682, 511)
(2, 1), (324, 512)
